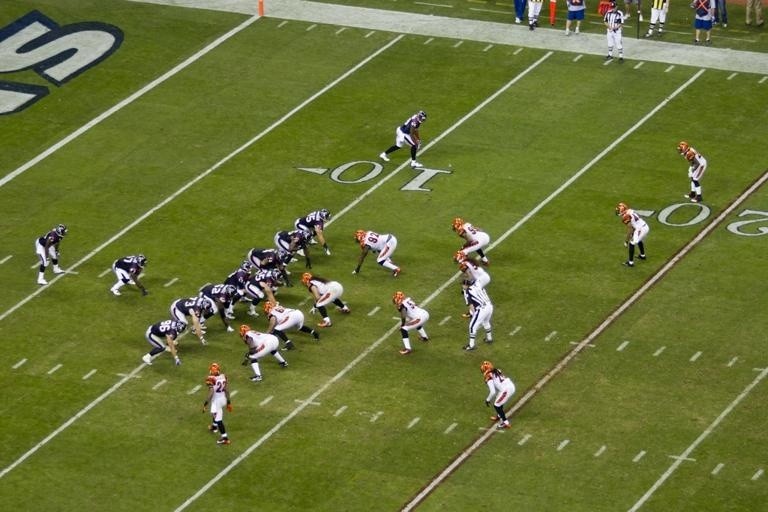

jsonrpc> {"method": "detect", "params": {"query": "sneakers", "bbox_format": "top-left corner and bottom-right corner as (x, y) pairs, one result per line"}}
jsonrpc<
(141, 343), (177, 366)
(207, 423), (230, 445)
(37, 265), (65, 285)
(694, 39), (710, 45)
(643, 31), (653, 38)
(463, 344), (477, 351)
(490, 415), (512, 430)
(398, 349), (410, 355)
(604, 54), (624, 64)
(634, 254), (645, 260)
(622, 261), (634, 267)
(109, 280), (136, 297)
(683, 190), (702, 202)
(461, 312), (471, 318)
(483, 336), (493, 345)
(316, 305), (349, 328)
(387, 257), (401, 277)
(481, 260), (490, 266)
(249, 360), (288, 382)
(565, 27), (580, 36)
(418, 335), (428, 341)
(281, 332), (319, 351)
(378, 151), (424, 168)
(515, 18), (539, 31)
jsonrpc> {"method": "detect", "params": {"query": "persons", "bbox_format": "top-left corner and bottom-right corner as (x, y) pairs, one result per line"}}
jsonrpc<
(393, 291), (430, 355)
(379, 110), (426, 167)
(481, 361), (516, 429)
(223, 246), (295, 316)
(274, 209), (333, 274)
(240, 325), (288, 381)
(616, 203), (650, 267)
(169, 283), (237, 347)
(202, 363), (232, 444)
(352, 230), (401, 277)
(301, 273), (349, 327)
(263, 301), (320, 350)
(110, 253), (148, 296)
(452, 218), (494, 351)
(142, 320), (188, 366)
(676, 142), (708, 202)
(514, 0), (764, 64)
(35, 223), (68, 285)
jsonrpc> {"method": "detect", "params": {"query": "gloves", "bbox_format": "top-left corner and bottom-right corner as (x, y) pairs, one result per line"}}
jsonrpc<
(203, 400), (208, 413)
(241, 353), (250, 367)
(139, 287), (149, 295)
(308, 306), (316, 315)
(352, 269), (360, 275)
(623, 240), (628, 249)
(486, 400), (490, 407)
(227, 400), (232, 413)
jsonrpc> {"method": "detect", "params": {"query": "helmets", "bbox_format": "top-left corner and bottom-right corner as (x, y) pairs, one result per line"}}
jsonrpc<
(450, 217), (464, 232)
(480, 359), (493, 373)
(615, 201), (628, 217)
(135, 253), (148, 270)
(302, 272), (311, 285)
(393, 290), (404, 306)
(55, 222), (68, 238)
(353, 229), (366, 241)
(677, 140), (687, 155)
(416, 109), (426, 124)
(263, 301), (274, 316)
(239, 324), (249, 338)
(208, 363), (221, 376)
(190, 208), (332, 347)
(452, 250), (467, 263)
